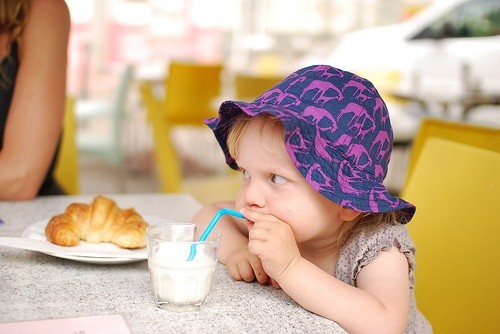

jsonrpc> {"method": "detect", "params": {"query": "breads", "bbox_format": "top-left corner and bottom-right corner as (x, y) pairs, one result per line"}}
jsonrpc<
(45, 195), (149, 250)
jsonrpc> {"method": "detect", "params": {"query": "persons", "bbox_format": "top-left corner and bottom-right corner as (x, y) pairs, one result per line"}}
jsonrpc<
(0, 0), (72, 202)
(188, 64), (435, 334)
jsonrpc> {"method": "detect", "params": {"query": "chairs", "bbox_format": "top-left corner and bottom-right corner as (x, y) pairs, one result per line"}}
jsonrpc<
(229, 72), (285, 102)
(54, 95), (79, 194)
(132, 61), (223, 172)
(397, 116), (500, 334)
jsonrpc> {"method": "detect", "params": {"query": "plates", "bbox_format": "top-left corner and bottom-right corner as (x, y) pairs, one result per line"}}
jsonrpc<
(22, 217), (147, 264)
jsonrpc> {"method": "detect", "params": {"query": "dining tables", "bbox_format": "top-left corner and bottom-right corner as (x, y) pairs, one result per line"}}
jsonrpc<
(0, 193), (351, 334)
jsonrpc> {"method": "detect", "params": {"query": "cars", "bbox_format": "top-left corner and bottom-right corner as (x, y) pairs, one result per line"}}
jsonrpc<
(299, 1), (499, 143)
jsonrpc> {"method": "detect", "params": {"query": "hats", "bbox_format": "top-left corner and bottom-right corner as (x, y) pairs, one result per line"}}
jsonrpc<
(201, 64), (416, 229)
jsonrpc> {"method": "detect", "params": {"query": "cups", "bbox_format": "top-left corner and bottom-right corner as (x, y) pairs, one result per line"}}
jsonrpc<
(146, 222), (221, 311)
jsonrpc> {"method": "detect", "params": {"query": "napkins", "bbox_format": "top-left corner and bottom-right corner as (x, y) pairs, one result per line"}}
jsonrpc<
(0, 236), (139, 260)
